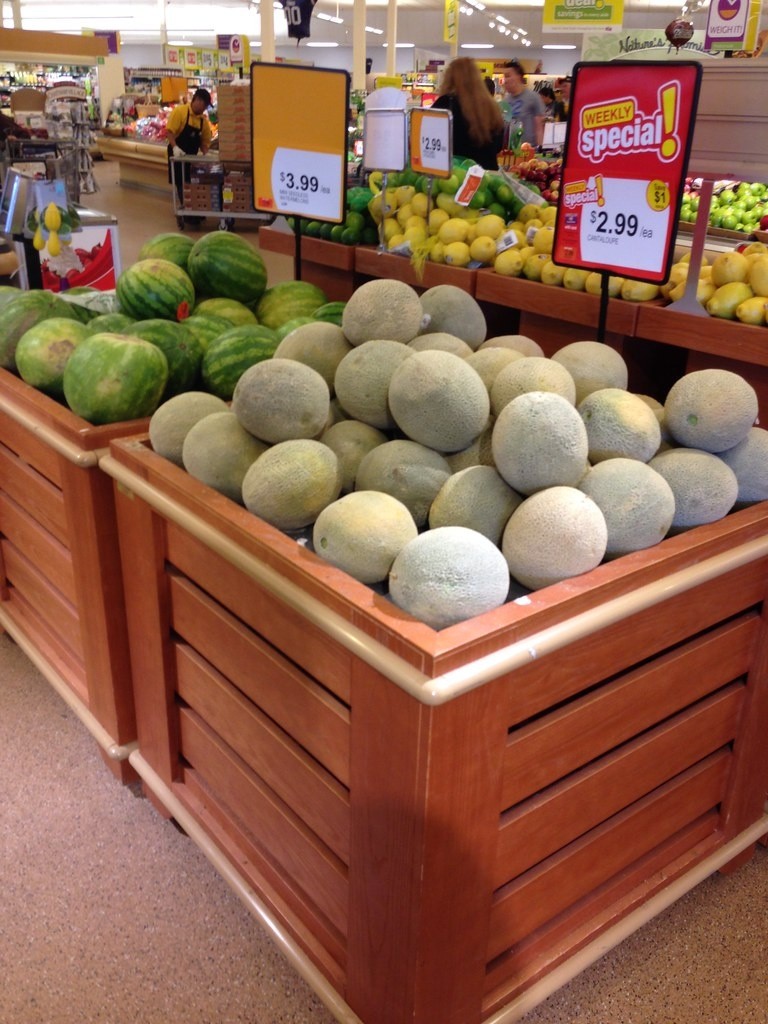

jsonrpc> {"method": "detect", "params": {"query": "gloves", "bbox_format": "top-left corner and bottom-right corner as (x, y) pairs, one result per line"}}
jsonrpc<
(173, 145), (186, 157)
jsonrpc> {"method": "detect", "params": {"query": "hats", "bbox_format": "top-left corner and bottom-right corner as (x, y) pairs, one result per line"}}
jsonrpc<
(559, 76), (572, 84)
(195, 89), (214, 109)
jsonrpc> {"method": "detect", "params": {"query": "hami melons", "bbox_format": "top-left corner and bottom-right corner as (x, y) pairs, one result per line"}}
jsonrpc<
(150, 278), (768, 636)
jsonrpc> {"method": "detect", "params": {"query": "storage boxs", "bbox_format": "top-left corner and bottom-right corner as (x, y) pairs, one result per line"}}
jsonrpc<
(188, 83), (252, 212)
(0, 367), (768, 1024)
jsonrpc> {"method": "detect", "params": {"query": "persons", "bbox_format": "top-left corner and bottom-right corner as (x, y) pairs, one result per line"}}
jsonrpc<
(163, 88), (215, 231)
(0, 109), (30, 191)
(429, 57), (505, 170)
(482, 59), (571, 147)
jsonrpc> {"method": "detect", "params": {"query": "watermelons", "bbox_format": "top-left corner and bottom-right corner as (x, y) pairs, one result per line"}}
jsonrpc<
(0, 231), (342, 426)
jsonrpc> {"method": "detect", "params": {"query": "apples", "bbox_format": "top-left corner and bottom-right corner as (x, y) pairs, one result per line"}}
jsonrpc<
(680, 178), (768, 234)
(508, 159), (563, 206)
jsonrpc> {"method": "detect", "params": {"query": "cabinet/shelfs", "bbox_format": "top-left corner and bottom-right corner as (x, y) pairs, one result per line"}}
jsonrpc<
(0, 58), (231, 154)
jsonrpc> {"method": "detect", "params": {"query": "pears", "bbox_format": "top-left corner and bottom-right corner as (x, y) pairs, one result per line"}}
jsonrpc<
(33, 202), (61, 256)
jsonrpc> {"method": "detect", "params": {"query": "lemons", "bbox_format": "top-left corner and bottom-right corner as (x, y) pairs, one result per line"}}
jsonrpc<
(494, 204), (768, 327)
(289, 159), (546, 266)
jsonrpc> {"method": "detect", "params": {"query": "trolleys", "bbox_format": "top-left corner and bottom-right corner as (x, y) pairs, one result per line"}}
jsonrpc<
(171, 156), (273, 231)
(6, 139), (80, 208)
